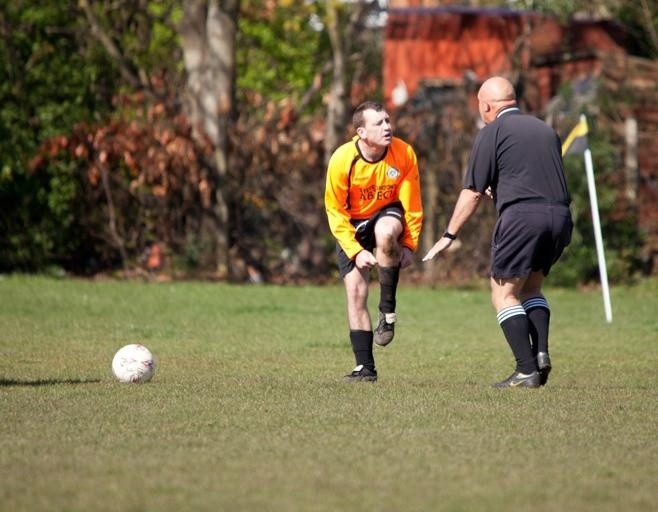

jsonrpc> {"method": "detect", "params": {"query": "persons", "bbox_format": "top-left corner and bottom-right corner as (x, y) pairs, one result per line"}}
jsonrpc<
(324, 99), (423, 381)
(420, 78), (573, 387)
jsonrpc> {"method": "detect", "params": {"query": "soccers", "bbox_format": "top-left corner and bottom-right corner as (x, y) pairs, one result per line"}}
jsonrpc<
(112, 345), (155, 384)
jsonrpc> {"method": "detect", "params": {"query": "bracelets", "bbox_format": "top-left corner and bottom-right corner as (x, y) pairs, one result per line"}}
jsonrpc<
(441, 230), (459, 240)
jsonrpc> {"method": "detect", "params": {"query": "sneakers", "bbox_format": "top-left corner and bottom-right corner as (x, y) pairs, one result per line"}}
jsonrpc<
(342, 363), (377, 383)
(374, 309), (397, 346)
(491, 367), (541, 389)
(535, 351), (552, 386)
